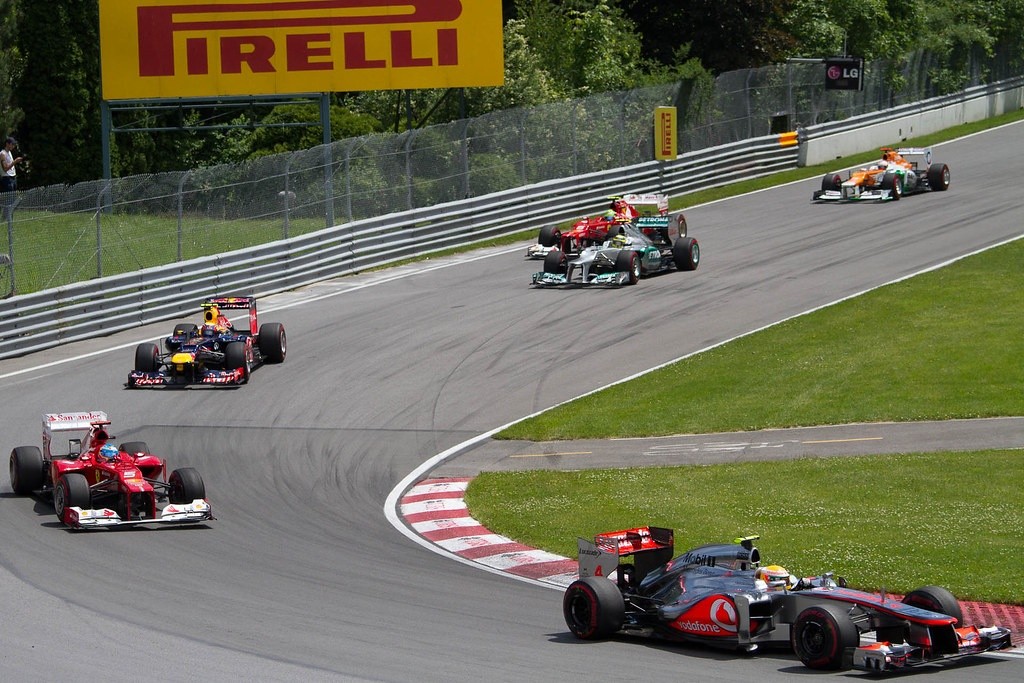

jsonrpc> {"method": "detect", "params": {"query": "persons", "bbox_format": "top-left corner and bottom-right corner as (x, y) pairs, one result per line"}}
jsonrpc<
(99, 444), (119, 474)
(877, 159), (888, 170)
(610, 234), (626, 249)
(1, 136), (22, 222)
(760, 564), (792, 593)
(200, 323), (219, 339)
(604, 209), (616, 220)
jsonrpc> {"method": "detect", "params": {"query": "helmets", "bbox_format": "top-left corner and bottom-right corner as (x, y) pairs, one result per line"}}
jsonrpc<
(202, 323), (217, 337)
(98, 443), (119, 461)
(612, 235), (626, 248)
(760, 565), (791, 590)
(877, 160), (889, 170)
(603, 209), (615, 221)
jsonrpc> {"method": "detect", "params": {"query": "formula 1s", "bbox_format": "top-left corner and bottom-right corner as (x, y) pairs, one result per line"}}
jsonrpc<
(563, 525), (1013, 676)
(808, 146), (952, 206)
(122, 294), (286, 388)
(8, 411), (220, 534)
(524, 193), (670, 259)
(530, 212), (702, 290)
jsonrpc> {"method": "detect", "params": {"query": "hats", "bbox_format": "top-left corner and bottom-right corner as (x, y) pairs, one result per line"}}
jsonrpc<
(6, 137), (18, 144)
